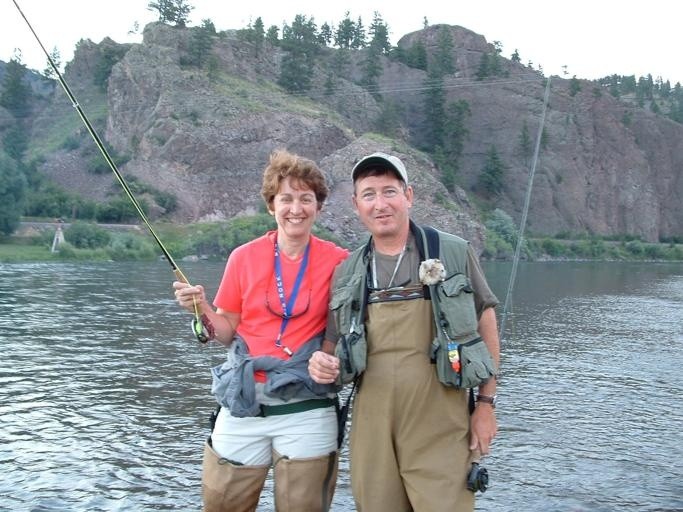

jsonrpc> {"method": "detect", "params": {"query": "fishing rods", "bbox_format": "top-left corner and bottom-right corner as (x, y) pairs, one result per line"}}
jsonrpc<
(467, 77), (552, 492)
(13, 1), (215, 344)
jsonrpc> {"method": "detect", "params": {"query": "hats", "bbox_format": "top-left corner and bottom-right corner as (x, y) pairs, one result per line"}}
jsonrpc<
(349, 150), (409, 186)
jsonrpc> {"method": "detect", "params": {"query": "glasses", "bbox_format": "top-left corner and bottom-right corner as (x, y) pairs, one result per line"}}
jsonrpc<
(264, 297), (311, 321)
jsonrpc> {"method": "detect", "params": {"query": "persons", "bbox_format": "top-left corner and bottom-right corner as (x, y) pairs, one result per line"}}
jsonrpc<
(174, 150), (353, 512)
(308, 152), (499, 512)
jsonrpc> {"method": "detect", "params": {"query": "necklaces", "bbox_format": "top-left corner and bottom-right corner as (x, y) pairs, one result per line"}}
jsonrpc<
(372, 242), (406, 292)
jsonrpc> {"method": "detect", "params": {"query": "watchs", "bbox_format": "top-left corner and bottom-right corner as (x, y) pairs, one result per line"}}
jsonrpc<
(477, 392), (498, 409)
